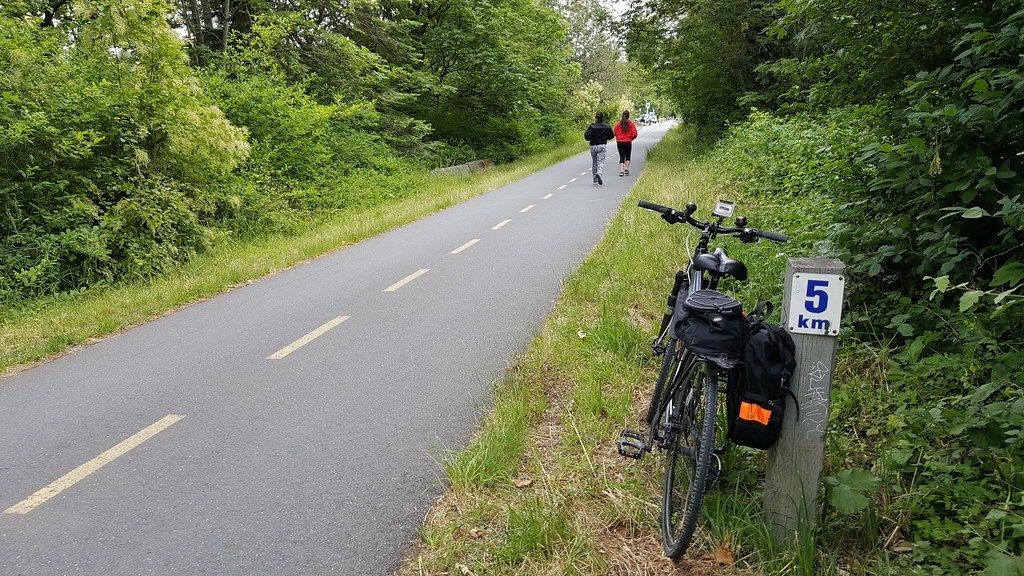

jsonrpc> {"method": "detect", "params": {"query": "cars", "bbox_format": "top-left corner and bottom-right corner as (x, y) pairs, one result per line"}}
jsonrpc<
(634, 113), (656, 124)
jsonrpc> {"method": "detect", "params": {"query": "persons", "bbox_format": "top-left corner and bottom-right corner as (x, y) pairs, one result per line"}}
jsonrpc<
(584, 112), (615, 186)
(614, 109), (638, 176)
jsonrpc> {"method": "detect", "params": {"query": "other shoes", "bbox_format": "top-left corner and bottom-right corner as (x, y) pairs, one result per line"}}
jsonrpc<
(619, 172), (623, 176)
(624, 170), (629, 175)
(596, 174), (603, 185)
(594, 180), (598, 185)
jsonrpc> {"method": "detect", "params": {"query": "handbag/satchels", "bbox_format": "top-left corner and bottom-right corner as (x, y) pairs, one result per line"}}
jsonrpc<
(674, 289), (748, 358)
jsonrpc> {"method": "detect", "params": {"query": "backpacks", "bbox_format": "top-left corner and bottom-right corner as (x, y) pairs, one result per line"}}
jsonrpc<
(724, 301), (799, 451)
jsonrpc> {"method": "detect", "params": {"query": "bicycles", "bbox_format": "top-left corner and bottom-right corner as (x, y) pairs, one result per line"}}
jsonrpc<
(613, 199), (791, 559)
